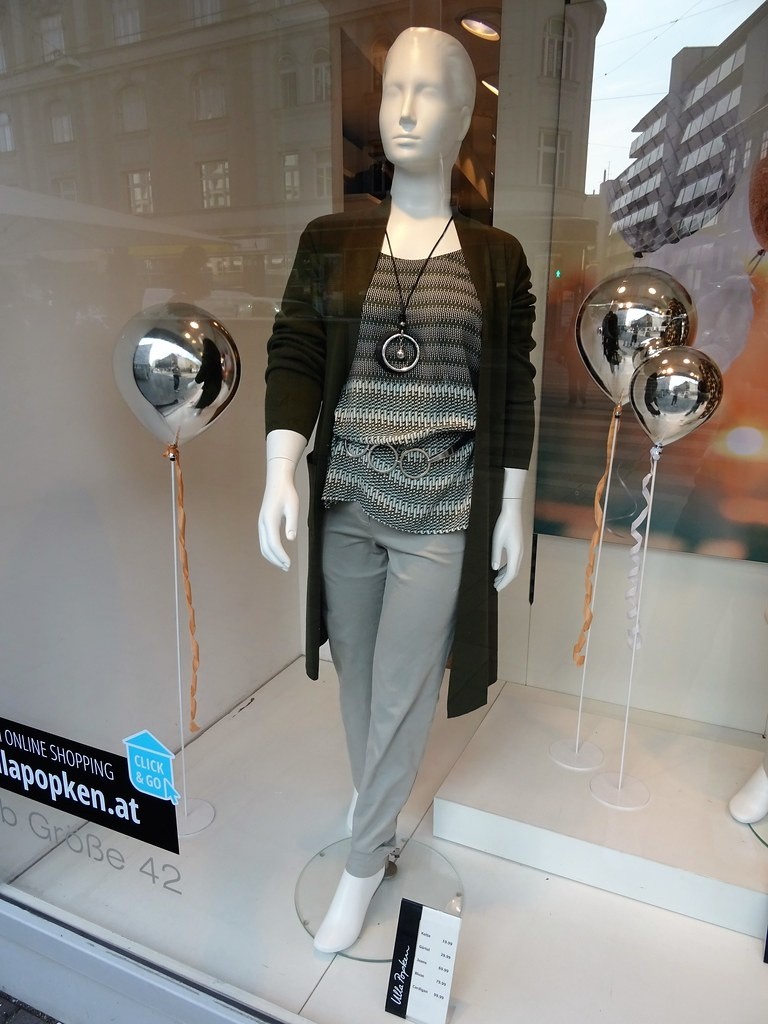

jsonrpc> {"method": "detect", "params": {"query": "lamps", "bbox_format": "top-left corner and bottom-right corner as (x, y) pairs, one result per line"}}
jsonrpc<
(461, 11), (502, 41)
(482, 71), (499, 95)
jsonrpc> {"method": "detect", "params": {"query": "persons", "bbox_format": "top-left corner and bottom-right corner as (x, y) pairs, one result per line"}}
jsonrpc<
(685, 376), (705, 417)
(602, 304), (623, 376)
(172, 362), (181, 393)
(644, 373), (661, 418)
(256, 27), (537, 951)
(194, 333), (223, 416)
(671, 390), (678, 407)
(629, 322), (638, 346)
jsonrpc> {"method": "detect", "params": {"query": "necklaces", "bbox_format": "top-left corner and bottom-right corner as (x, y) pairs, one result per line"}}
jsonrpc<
(375, 214), (455, 377)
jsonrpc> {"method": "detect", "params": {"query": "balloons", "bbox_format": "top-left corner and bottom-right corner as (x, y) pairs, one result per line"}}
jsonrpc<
(632, 336), (668, 370)
(112, 301), (241, 447)
(574, 266), (699, 409)
(628, 346), (724, 447)
